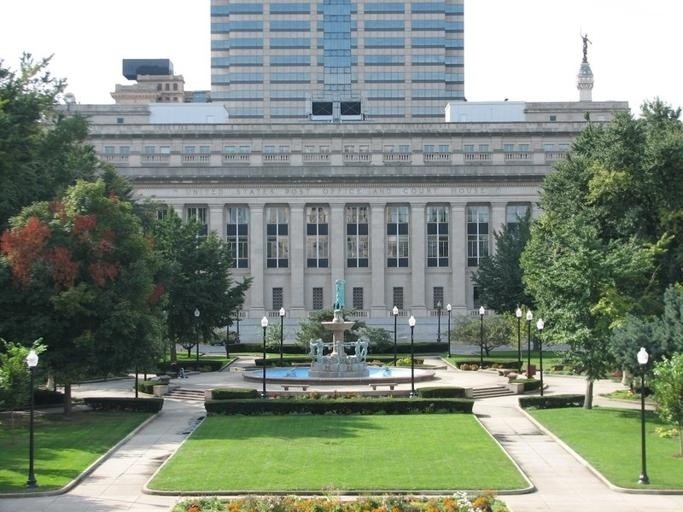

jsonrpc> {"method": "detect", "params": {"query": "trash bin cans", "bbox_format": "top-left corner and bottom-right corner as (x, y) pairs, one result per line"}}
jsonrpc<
(529, 365), (536, 375)
(410, 392), (418, 398)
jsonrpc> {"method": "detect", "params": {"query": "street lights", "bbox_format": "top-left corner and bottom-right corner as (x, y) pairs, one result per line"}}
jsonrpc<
(278, 307), (286, 366)
(408, 313), (418, 398)
(25, 348), (39, 489)
(260, 315), (270, 400)
(236, 302), (240, 344)
(446, 304), (454, 358)
(391, 306), (399, 367)
(194, 307), (202, 370)
(161, 310), (169, 371)
(436, 300), (442, 344)
(515, 307), (546, 396)
(477, 305), (485, 370)
(636, 345), (649, 486)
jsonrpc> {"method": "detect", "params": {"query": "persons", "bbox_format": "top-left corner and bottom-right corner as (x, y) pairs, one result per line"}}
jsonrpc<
(355, 339), (368, 362)
(333, 280), (345, 310)
(308, 338), (323, 363)
